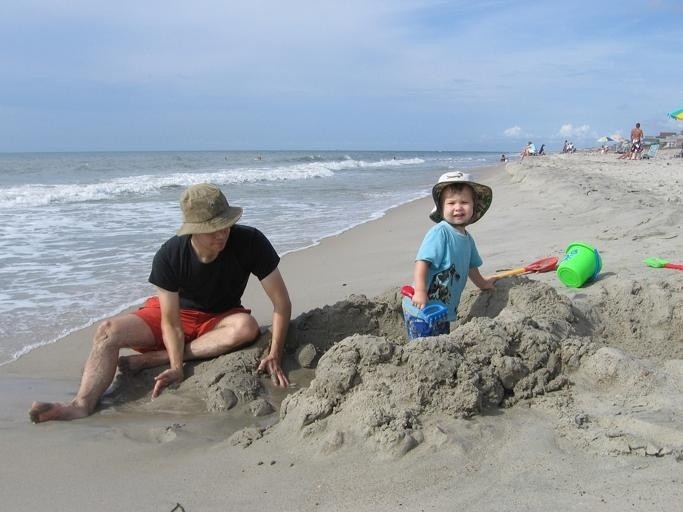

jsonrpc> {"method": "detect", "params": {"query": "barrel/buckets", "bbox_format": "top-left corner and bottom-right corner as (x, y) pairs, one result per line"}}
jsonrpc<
(556, 243), (603, 287)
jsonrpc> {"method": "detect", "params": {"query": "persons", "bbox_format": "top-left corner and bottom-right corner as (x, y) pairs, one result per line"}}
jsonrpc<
(618, 122), (644, 159)
(413, 170), (499, 337)
(498, 138), (575, 162)
(26, 184), (291, 425)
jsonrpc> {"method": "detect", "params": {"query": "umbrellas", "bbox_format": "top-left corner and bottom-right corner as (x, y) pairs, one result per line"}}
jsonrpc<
(598, 136), (613, 145)
(667, 107), (683, 121)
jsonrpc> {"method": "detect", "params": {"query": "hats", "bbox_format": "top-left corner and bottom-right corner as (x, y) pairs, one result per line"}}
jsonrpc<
(430, 172), (492, 225)
(177, 186), (242, 237)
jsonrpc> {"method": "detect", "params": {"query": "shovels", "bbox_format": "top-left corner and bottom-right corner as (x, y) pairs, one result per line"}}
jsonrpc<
(642, 257), (683, 270)
(489, 256), (561, 283)
